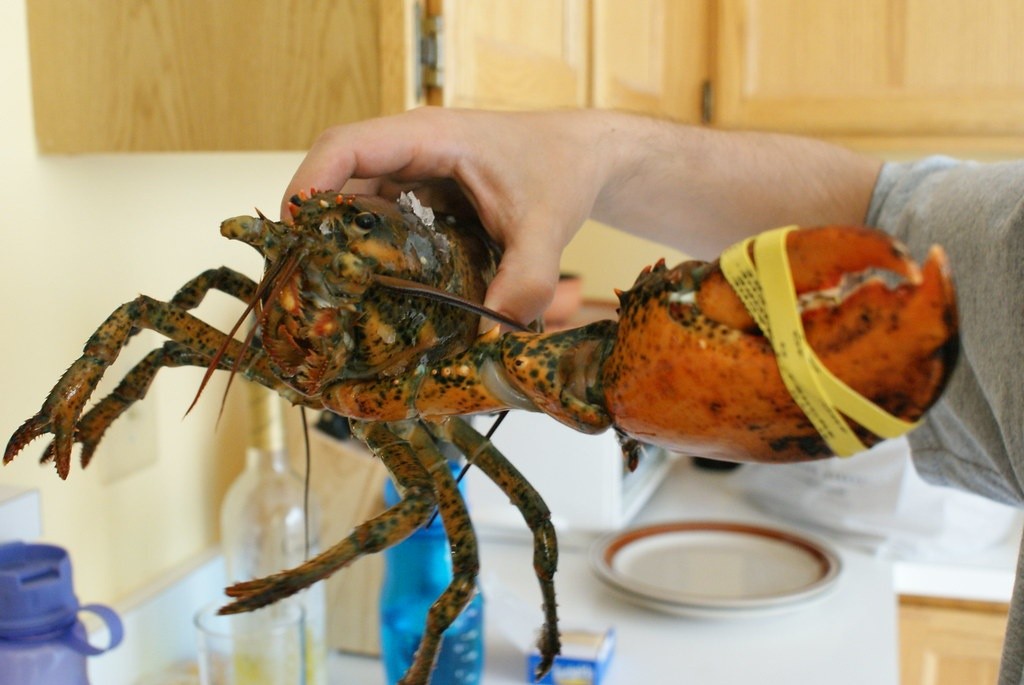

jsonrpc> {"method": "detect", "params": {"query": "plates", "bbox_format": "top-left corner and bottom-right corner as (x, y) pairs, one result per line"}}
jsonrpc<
(591, 522), (842, 608)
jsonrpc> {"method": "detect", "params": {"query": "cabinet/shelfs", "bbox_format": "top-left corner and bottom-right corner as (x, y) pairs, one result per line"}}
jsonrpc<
(24, 0), (1024, 162)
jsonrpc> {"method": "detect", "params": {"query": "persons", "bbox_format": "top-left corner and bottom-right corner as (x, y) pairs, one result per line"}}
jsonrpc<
(281, 105), (1023, 685)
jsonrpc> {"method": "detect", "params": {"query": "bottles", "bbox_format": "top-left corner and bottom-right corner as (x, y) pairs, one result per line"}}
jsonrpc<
(379, 445), (483, 685)
(0, 541), (123, 685)
(220, 446), (325, 684)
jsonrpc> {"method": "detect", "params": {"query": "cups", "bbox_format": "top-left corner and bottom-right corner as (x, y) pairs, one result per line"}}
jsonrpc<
(195, 600), (305, 685)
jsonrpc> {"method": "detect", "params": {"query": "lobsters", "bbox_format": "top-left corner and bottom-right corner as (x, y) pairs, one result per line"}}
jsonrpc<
(1, 178), (962, 684)
